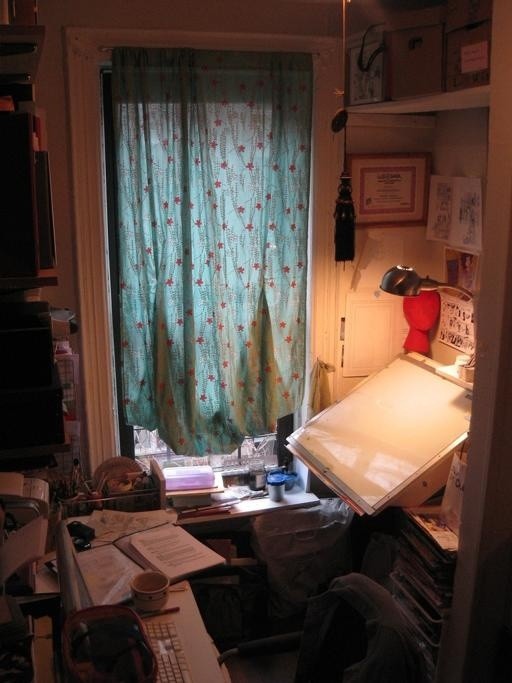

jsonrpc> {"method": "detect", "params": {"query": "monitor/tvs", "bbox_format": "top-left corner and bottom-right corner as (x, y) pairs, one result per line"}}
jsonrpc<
(55, 520), (95, 618)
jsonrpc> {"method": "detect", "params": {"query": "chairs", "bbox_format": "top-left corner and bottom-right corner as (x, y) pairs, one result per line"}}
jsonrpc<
(217, 572), (422, 681)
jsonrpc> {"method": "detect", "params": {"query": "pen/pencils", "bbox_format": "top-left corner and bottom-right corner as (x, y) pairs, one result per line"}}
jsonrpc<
(56, 458), (80, 498)
(137, 607), (181, 618)
(170, 588), (185, 592)
(81, 472), (110, 492)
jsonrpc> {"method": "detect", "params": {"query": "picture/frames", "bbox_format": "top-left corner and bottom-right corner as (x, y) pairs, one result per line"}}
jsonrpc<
(345, 152), (434, 230)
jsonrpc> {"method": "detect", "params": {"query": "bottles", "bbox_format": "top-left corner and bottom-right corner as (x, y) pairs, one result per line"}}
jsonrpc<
(248, 458), (267, 491)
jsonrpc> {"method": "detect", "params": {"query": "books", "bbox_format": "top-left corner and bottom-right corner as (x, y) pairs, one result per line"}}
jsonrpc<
(165, 487), (240, 517)
(44, 522), (225, 606)
(150, 459), (166, 510)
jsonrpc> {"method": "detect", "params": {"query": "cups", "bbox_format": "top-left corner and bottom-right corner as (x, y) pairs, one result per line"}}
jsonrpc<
(266, 473), (286, 502)
(130, 571), (170, 612)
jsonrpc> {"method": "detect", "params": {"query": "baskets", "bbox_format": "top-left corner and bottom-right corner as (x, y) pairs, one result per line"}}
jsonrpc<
(61, 605), (158, 683)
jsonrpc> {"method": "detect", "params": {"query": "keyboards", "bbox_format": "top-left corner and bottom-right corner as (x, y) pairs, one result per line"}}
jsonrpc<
(142, 619), (194, 683)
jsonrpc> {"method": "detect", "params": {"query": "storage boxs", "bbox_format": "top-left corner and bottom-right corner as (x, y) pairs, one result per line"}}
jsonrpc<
(344, 1), (491, 108)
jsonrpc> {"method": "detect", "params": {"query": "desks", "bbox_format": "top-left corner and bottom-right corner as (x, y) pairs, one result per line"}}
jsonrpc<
(59, 462), (319, 683)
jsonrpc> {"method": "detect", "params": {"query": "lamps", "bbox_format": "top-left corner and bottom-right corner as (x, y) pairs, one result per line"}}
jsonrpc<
(330, 109), (356, 263)
(379, 265), (475, 317)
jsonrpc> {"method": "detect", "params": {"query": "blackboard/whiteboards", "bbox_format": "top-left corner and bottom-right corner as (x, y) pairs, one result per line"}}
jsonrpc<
(285, 354), (473, 516)
